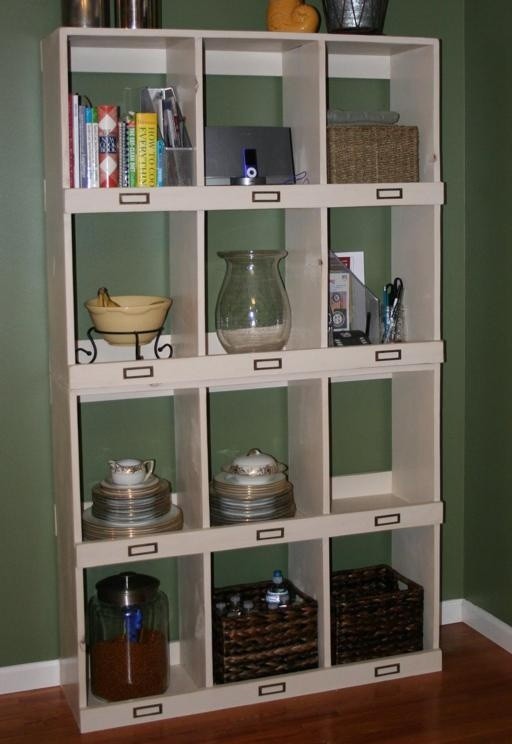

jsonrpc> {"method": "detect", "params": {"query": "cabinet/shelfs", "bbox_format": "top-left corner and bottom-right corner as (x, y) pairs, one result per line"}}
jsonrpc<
(41, 27), (443, 734)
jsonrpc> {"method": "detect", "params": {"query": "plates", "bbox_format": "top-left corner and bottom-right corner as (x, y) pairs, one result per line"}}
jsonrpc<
(82, 473), (183, 541)
(209, 471), (297, 526)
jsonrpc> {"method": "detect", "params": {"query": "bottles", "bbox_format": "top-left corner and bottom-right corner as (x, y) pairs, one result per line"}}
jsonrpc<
(214, 592), (254, 618)
(89, 572), (170, 701)
(267, 570), (289, 607)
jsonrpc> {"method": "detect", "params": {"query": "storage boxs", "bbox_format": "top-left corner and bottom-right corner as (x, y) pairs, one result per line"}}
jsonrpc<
(165, 148), (192, 186)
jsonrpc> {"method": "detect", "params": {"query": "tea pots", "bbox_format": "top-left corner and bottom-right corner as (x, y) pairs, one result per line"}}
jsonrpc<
(107, 458), (155, 485)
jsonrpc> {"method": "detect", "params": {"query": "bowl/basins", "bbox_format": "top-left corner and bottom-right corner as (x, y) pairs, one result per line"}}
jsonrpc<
(84, 295), (173, 347)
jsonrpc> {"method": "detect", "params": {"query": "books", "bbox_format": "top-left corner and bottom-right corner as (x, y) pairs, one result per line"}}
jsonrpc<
(67, 94), (158, 190)
